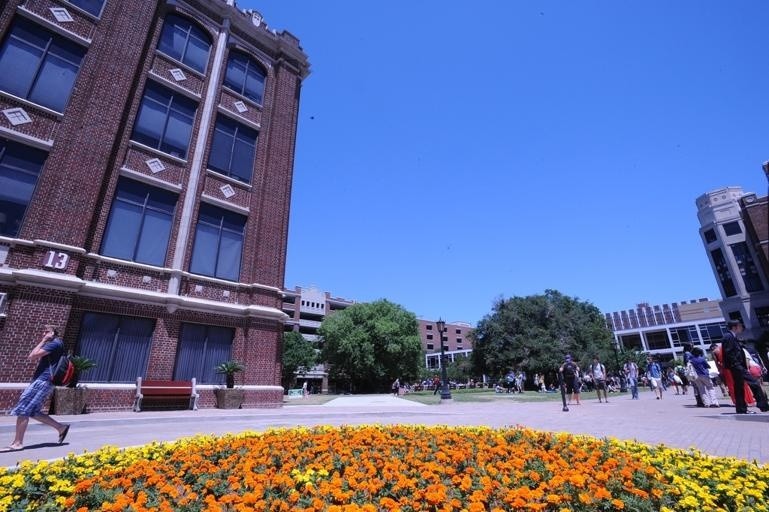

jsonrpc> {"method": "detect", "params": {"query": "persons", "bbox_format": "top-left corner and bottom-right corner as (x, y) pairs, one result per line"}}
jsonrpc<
(589, 355), (608, 403)
(623, 357), (639, 400)
(646, 356), (663, 400)
(559, 354), (582, 404)
(0, 323), (71, 453)
(303, 378), (322, 400)
(740, 341), (769, 402)
(720, 318), (768, 415)
(709, 343), (730, 397)
(683, 341), (706, 407)
(392, 374), (475, 397)
(640, 362), (692, 395)
(583, 374), (621, 393)
(689, 347), (720, 407)
(495, 368), (556, 394)
(741, 347), (762, 385)
(716, 344), (758, 408)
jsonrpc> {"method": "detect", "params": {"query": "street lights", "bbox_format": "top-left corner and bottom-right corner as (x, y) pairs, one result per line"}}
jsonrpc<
(610, 339), (628, 392)
(436, 315), (452, 399)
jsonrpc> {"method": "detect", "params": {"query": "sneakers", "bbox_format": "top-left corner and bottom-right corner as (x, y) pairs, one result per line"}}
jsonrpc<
(600, 400), (608, 403)
(697, 402), (718, 408)
(632, 395), (638, 400)
(567, 401), (580, 405)
(657, 397), (663, 400)
(736, 409), (756, 414)
(675, 391), (686, 395)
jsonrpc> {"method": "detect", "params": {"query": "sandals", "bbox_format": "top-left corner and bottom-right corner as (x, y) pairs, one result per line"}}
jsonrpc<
(57, 424), (70, 446)
(0, 442), (25, 453)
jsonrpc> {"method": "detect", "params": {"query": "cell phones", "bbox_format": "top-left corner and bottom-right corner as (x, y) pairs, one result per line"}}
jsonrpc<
(54, 329), (59, 335)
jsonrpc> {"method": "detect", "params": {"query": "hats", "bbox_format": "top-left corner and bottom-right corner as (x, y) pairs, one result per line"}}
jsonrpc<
(565, 355), (574, 360)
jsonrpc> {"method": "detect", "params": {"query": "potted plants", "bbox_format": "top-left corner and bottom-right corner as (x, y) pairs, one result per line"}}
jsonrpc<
(216, 361), (243, 388)
(68, 356), (98, 388)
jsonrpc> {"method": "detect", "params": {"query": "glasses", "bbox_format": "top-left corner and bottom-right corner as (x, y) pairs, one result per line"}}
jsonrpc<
(43, 330), (50, 334)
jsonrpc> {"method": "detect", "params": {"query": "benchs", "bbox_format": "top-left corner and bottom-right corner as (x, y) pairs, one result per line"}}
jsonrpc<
(132, 377), (200, 411)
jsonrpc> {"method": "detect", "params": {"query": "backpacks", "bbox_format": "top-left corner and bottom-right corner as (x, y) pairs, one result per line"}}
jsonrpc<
(684, 359), (699, 382)
(677, 367), (685, 380)
(563, 362), (576, 381)
(49, 340), (75, 386)
